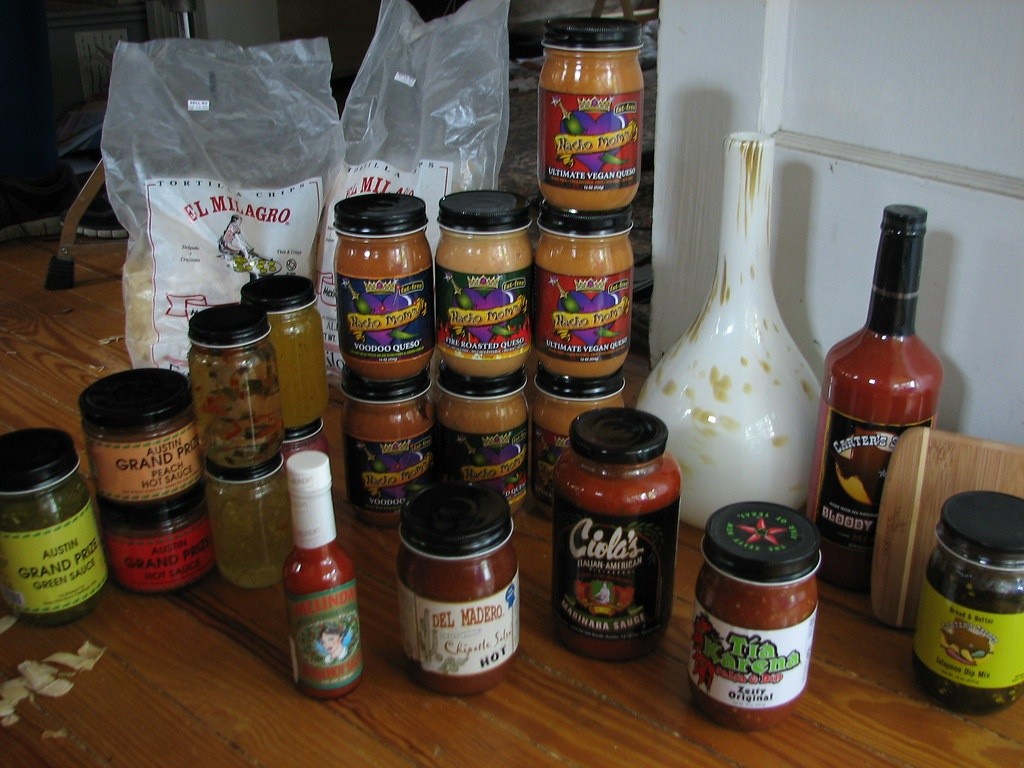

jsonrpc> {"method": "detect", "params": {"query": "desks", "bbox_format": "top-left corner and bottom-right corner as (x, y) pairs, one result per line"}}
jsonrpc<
(2, 233), (1024, 768)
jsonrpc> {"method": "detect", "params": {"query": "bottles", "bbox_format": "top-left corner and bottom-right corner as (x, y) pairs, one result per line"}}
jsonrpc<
(804, 201), (943, 599)
(198, 451), (302, 589)
(187, 302), (283, 470)
(277, 449), (365, 699)
(238, 273), (328, 432)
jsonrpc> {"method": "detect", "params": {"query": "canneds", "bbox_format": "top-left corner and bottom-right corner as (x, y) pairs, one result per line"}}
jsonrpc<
(435, 188), (634, 528)
(1, 427), (109, 626)
(80, 368), (204, 512)
(337, 364), (433, 528)
(551, 406), (682, 663)
(687, 501), (823, 729)
(910, 491), (1024, 714)
(239, 274), (329, 429)
(187, 301), (283, 469)
(333, 190), (436, 379)
(534, 15), (646, 209)
(204, 456), (296, 590)
(96, 485), (215, 598)
(395, 484), (522, 696)
